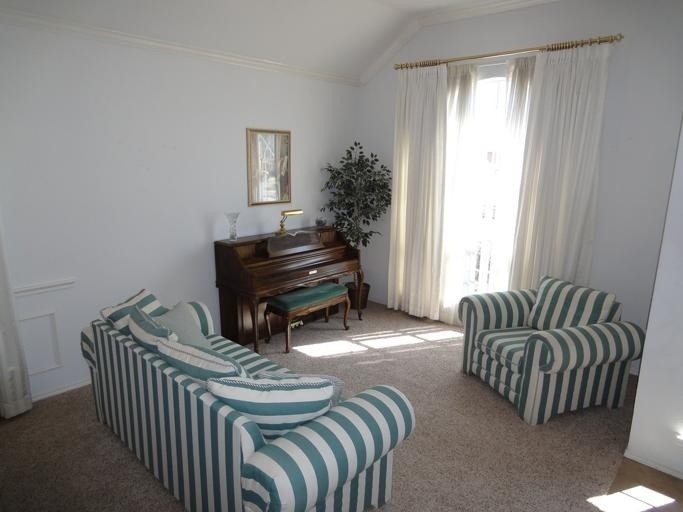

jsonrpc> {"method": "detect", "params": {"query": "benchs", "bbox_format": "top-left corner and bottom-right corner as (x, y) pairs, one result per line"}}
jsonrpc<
(263, 281), (352, 355)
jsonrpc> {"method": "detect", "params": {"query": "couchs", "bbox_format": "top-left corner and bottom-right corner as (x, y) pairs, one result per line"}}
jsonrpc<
(78, 297), (419, 511)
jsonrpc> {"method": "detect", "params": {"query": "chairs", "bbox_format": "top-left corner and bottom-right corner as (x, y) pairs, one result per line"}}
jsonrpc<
(455, 284), (647, 426)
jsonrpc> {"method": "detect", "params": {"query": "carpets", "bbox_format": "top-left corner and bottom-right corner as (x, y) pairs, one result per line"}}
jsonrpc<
(0, 300), (638, 511)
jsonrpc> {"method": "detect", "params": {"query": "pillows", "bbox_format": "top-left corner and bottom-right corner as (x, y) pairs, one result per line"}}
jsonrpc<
(202, 374), (335, 443)
(258, 369), (346, 408)
(96, 285), (169, 337)
(126, 302), (179, 354)
(149, 299), (212, 349)
(153, 333), (257, 390)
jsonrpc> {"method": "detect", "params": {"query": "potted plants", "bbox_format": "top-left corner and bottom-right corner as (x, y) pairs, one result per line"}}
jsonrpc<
(315, 134), (392, 311)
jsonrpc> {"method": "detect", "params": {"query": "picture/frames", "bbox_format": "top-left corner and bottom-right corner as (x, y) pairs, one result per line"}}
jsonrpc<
(245, 127), (291, 207)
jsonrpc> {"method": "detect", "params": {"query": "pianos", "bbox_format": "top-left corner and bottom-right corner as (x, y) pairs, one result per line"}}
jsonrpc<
(214, 226), (363, 354)
